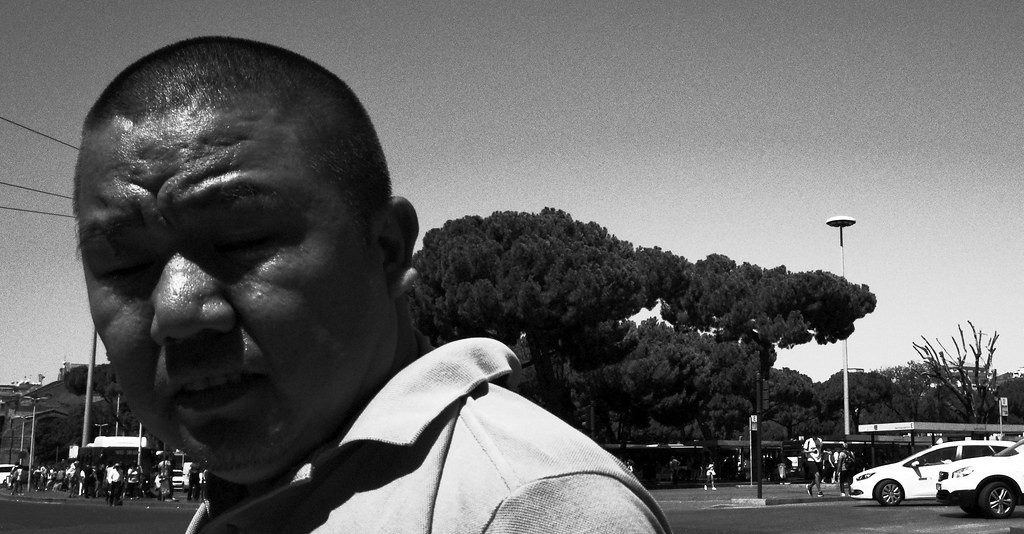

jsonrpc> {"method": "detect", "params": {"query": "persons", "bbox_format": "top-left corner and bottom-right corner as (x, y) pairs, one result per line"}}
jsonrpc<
(836, 443), (857, 497)
(744, 452), (793, 485)
(667, 455), (696, 483)
(822, 445), (868, 485)
(75, 35), (678, 534)
(802, 431), (825, 498)
(703, 456), (717, 491)
(186, 458), (201, 502)
(8, 453), (177, 507)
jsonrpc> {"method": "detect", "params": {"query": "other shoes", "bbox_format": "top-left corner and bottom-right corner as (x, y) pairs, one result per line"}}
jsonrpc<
(712, 487), (717, 491)
(806, 484), (813, 496)
(841, 493), (846, 497)
(704, 485), (708, 491)
(817, 491), (824, 497)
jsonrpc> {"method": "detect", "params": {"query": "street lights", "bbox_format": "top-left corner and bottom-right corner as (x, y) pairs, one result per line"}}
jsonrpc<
(23, 395), (48, 492)
(824, 215), (856, 436)
(94, 423), (109, 436)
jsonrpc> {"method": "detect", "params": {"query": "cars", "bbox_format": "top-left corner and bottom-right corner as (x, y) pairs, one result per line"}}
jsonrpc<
(170, 469), (184, 490)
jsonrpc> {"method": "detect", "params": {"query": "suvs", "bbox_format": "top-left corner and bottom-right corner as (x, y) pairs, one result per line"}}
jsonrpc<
(848, 440), (1024, 520)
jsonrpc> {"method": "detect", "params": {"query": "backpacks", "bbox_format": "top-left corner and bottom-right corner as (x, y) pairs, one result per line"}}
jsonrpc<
(841, 450), (855, 470)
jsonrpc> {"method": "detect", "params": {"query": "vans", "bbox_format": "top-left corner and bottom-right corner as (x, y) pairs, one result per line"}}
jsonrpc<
(0, 463), (18, 488)
